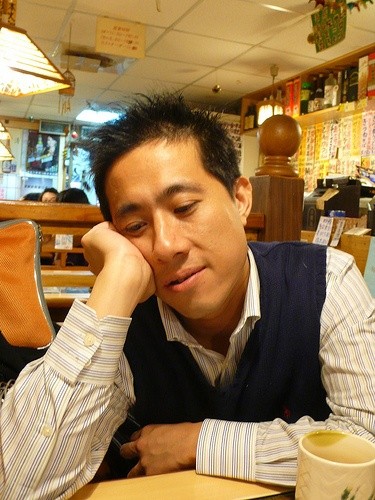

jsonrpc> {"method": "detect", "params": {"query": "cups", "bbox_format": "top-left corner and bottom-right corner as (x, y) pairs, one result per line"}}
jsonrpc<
(294, 430), (375, 500)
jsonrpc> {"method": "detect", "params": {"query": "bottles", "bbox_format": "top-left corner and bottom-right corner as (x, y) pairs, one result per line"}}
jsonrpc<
(300, 62), (359, 114)
(243, 87), (285, 130)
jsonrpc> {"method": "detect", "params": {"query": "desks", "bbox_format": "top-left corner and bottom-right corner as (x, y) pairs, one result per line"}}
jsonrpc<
(68, 469), (297, 500)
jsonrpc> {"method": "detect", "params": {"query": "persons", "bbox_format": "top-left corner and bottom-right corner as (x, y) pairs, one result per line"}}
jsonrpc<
(1, 89), (375, 500)
(22, 188), (90, 266)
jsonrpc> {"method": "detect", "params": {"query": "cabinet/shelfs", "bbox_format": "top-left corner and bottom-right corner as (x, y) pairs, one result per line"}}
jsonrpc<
(239, 41), (375, 136)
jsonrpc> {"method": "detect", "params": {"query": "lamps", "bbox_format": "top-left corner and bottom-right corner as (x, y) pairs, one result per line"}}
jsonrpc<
(257, 64), (285, 127)
(0, 0), (72, 98)
(61, 50), (115, 74)
(75, 100), (130, 123)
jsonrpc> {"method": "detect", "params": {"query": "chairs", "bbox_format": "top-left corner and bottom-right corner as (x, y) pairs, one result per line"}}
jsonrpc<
(0, 218), (56, 351)
